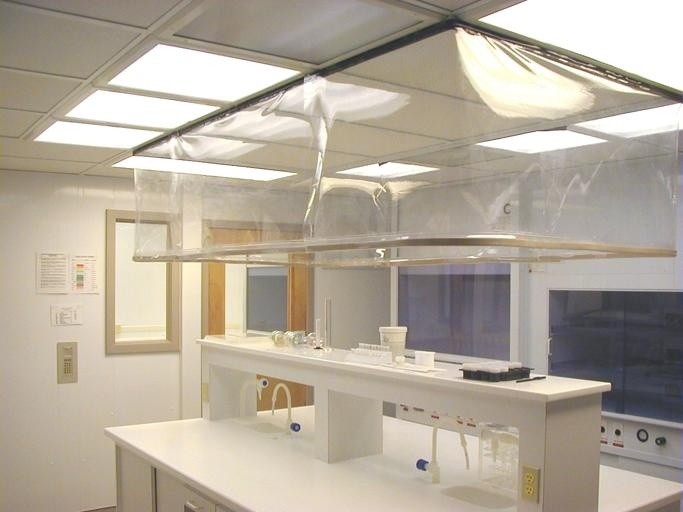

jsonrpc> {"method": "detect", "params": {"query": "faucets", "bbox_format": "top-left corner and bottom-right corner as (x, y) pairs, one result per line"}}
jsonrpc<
(271, 382), (301, 434)
(239, 377), (269, 418)
(416, 415), (470, 484)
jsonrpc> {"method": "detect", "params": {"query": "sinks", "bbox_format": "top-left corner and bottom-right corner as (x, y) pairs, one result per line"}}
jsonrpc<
(245, 421), (290, 438)
(441, 485), (516, 510)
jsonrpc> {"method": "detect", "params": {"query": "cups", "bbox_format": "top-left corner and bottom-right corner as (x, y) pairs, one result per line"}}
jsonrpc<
(414, 350), (435, 367)
(461, 361), (522, 373)
(350, 343), (393, 367)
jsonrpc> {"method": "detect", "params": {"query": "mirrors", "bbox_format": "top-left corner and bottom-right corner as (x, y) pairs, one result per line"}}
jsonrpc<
(199, 217), (318, 415)
(105, 207), (184, 358)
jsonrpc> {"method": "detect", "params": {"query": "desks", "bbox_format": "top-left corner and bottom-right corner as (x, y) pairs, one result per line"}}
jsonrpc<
(103, 403), (682, 512)
(193, 333), (612, 512)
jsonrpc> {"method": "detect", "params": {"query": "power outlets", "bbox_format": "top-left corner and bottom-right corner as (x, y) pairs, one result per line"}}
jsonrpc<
(519, 464), (541, 505)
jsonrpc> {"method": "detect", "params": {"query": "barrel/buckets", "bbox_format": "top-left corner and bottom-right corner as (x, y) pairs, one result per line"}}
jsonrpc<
(378, 325), (408, 362)
